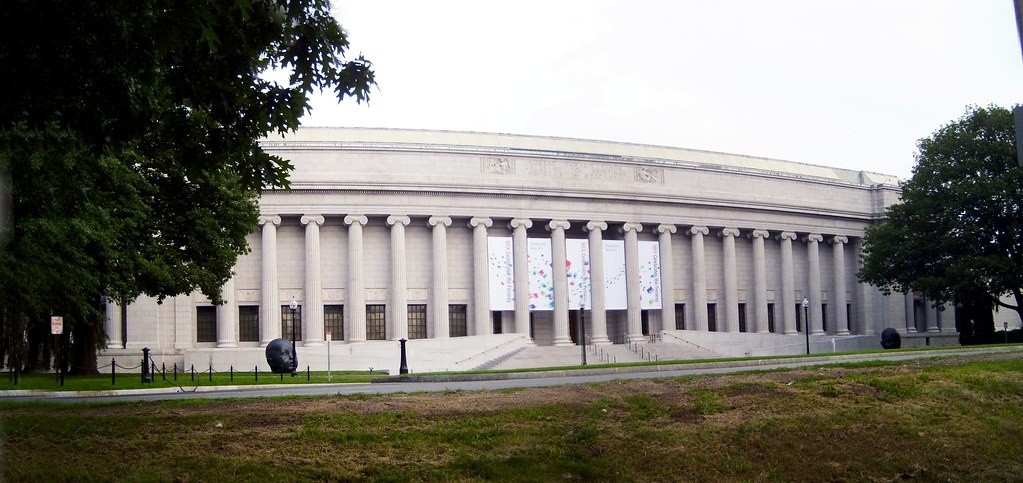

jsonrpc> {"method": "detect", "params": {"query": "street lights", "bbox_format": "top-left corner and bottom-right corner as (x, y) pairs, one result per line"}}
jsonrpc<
(802, 295), (810, 355)
(290, 295), (298, 377)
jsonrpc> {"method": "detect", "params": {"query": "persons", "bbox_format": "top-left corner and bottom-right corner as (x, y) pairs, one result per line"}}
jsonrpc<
(265, 338), (298, 373)
